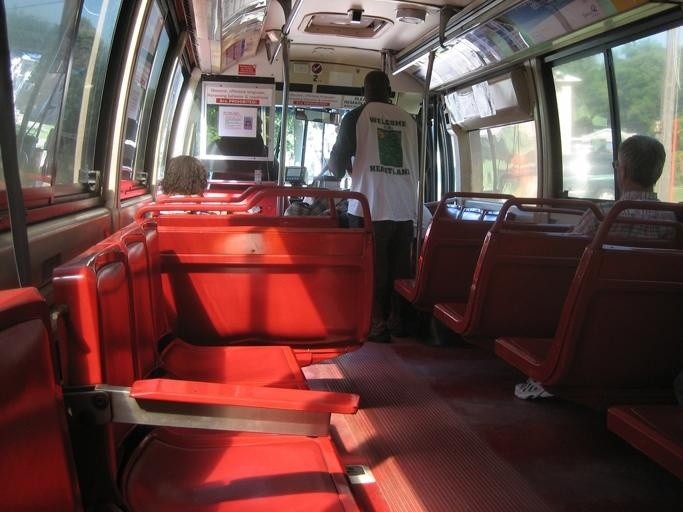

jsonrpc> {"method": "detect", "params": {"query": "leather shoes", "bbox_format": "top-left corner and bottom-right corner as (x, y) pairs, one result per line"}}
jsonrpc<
(369, 331), (392, 341)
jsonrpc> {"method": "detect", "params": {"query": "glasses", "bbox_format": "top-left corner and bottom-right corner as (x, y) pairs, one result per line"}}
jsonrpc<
(612, 160), (621, 168)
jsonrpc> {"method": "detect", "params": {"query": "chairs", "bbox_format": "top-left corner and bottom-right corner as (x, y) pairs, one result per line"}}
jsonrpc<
(491, 198), (682, 407)
(603, 397), (681, 475)
(394, 194), (589, 349)
(426, 193), (681, 359)
(4, 179), (392, 509)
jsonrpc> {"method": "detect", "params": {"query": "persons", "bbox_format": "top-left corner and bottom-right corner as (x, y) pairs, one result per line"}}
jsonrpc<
(513, 134), (677, 401)
(159, 156), (223, 216)
(280, 202), (349, 227)
(326, 70), (429, 342)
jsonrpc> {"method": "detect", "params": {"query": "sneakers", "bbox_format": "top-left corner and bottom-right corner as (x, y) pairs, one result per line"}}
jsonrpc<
(515, 378), (556, 399)
